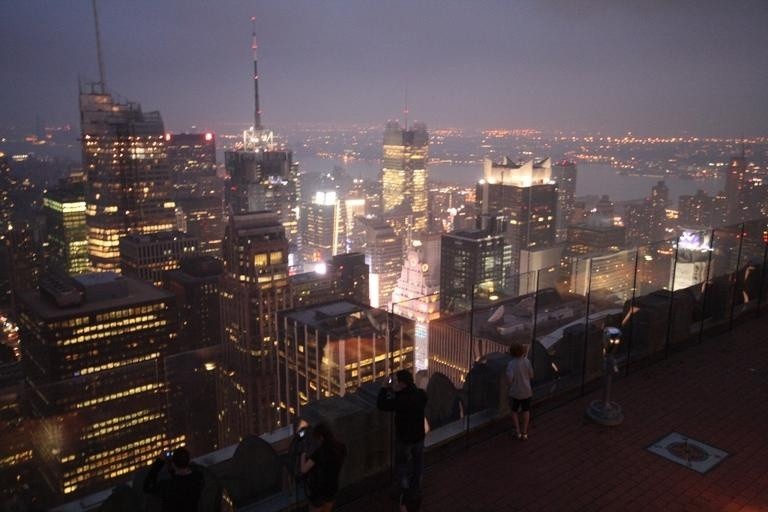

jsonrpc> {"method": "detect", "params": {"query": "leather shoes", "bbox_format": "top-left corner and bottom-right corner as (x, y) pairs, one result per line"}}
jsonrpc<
(522, 432), (528, 440)
(511, 432), (522, 441)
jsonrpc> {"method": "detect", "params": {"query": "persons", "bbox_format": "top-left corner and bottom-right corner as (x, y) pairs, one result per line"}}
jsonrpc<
(300, 421), (348, 512)
(376, 369), (428, 502)
(505, 342), (534, 441)
(399, 487), (423, 512)
(143, 446), (205, 512)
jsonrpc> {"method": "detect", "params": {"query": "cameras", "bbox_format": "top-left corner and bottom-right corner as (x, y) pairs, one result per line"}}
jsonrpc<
(296, 426), (310, 441)
